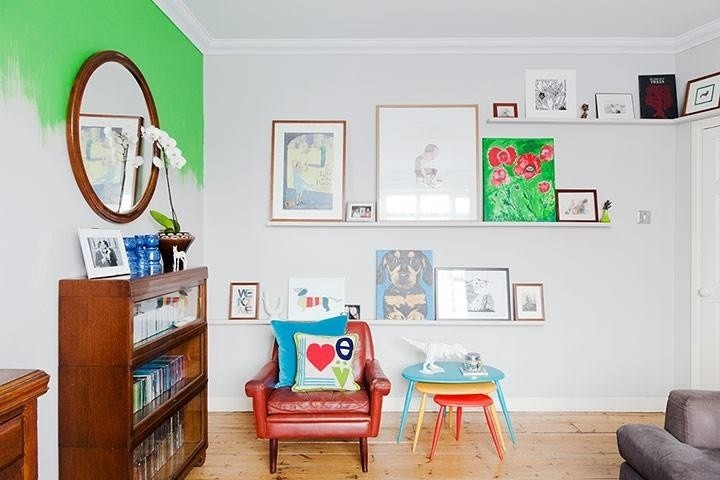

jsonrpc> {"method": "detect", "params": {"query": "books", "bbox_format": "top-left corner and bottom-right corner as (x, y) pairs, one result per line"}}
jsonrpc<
(133, 284), (201, 479)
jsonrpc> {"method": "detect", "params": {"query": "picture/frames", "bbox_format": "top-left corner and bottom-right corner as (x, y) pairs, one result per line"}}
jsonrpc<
(434, 267), (512, 320)
(525, 69), (577, 119)
(513, 283), (545, 321)
(375, 103), (483, 222)
(680, 72), (720, 117)
(595, 92), (635, 119)
(268, 119), (346, 222)
(493, 103), (518, 117)
(227, 281), (260, 320)
(555, 189), (598, 222)
(77, 228), (131, 280)
(80, 113), (144, 207)
(345, 201), (375, 222)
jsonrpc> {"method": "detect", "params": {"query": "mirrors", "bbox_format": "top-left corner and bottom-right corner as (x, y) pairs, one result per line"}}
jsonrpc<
(66, 50), (160, 223)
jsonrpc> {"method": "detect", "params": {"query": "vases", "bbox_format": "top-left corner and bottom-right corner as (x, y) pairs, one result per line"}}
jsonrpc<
(159, 233), (195, 266)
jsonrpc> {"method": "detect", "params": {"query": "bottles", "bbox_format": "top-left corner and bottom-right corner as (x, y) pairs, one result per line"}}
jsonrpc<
(463, 351), (484, 375)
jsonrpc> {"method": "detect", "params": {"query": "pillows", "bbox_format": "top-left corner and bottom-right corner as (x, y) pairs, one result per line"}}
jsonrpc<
(269, 315), (348, 388)
(290, 332), (361, 392)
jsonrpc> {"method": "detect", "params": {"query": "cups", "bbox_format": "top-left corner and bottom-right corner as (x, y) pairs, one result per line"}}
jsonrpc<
(122, 234), (163, 281)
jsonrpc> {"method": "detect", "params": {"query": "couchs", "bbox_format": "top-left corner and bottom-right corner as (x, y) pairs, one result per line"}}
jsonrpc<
(614, 389), (720, 480)
(244, 320), (391, 473)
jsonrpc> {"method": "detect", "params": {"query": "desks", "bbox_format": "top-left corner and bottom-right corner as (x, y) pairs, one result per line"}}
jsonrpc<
(0, 369), (51, 480)
(397, 361), (517, 444)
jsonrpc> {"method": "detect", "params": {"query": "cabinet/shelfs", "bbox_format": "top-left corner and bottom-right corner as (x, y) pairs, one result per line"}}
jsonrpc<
(58, 267), (209, 480)
(209, 109), (718, 326)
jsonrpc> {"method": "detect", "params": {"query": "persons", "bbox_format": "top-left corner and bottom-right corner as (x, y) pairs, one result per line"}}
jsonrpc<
(103, 240), (118, 267)
(362, 207), (371, 218)
(95, 241), (106, 267)
(349, 306), (360, 320)
(352, 207), (361, 218)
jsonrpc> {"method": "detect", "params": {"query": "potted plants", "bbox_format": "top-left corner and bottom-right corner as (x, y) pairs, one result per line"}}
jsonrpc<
(600, 199), (612, 223)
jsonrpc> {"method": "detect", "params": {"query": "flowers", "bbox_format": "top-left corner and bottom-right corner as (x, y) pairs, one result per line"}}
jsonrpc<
(138, 125), (188, 235)
(100, 123), (144, 212)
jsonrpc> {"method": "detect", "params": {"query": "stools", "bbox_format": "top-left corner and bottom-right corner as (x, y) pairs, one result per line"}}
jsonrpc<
(429, 395), (503, 461)
(411, 382), (507, 452)
